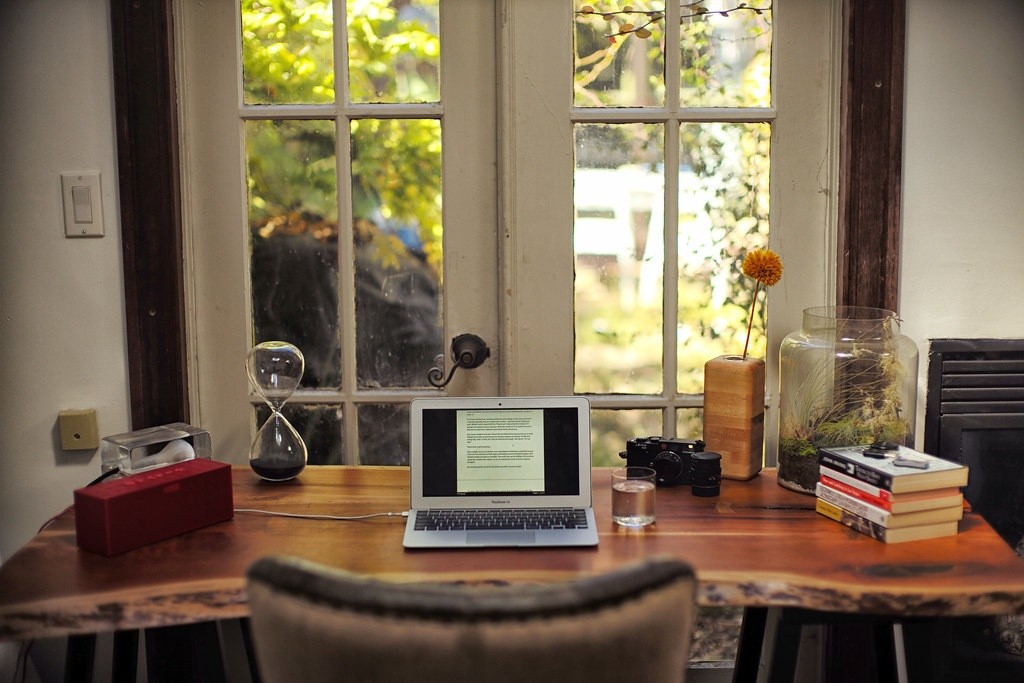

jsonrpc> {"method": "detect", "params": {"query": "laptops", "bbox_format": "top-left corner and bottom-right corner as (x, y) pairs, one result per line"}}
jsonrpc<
(402, 396), (599, 547)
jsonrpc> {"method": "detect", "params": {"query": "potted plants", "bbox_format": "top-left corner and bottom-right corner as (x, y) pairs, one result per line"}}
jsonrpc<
(776, 306), (919, 494)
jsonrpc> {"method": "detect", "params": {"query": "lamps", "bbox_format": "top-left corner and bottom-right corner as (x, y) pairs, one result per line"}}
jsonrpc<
(39, 422), (212, 534)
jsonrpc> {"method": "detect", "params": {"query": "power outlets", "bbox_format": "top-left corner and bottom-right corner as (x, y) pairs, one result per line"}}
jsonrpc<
(59, 409), (98, 450)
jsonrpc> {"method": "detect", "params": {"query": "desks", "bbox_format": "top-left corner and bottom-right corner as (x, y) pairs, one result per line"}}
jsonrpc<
(0, 469), (1024, 683)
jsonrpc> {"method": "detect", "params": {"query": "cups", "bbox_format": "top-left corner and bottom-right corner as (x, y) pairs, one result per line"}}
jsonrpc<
(611, 467), (656, 527)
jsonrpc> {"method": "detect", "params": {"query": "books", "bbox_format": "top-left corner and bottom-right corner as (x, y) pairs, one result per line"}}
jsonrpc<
(816, 444), (968, 543)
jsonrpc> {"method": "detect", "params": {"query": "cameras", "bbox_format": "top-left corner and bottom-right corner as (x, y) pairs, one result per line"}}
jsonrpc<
(626, 436), (706, 486)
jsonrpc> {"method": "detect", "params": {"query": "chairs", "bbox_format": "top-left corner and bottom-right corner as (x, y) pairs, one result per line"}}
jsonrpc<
(245, 553), (699, 683)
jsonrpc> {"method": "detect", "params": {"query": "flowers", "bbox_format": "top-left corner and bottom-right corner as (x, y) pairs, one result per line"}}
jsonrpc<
(742, 248), (783, 360)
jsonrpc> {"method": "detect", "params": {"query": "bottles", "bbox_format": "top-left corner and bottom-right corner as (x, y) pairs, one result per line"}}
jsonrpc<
(776, 306), (919, 496)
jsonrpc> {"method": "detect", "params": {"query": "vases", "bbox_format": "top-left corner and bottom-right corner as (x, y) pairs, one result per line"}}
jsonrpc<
(703, 355), (765, 480)
